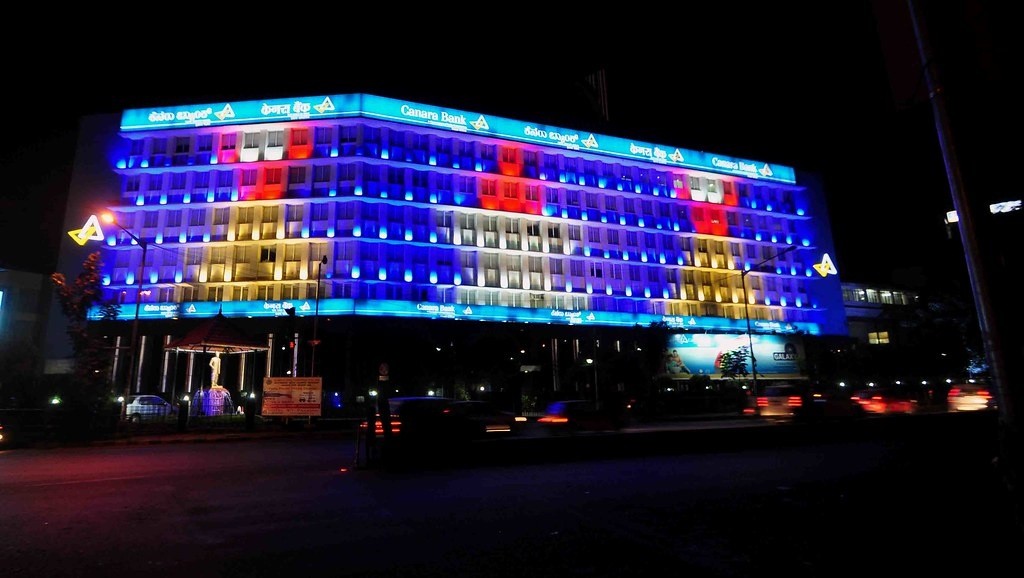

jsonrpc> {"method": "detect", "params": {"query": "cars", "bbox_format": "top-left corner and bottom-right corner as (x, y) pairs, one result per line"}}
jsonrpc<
(113, 394), (175, 424)
(366, 395), (526, 445)
(756, 378), (994, 419)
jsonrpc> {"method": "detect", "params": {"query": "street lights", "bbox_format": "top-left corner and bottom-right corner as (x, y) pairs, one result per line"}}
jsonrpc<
(740, 244), (797, 399)
(97, 208), (149, 423)
(310, 254), (328, 377)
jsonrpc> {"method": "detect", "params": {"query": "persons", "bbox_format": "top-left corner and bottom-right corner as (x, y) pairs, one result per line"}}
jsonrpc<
(209, 352), (221, 386)
